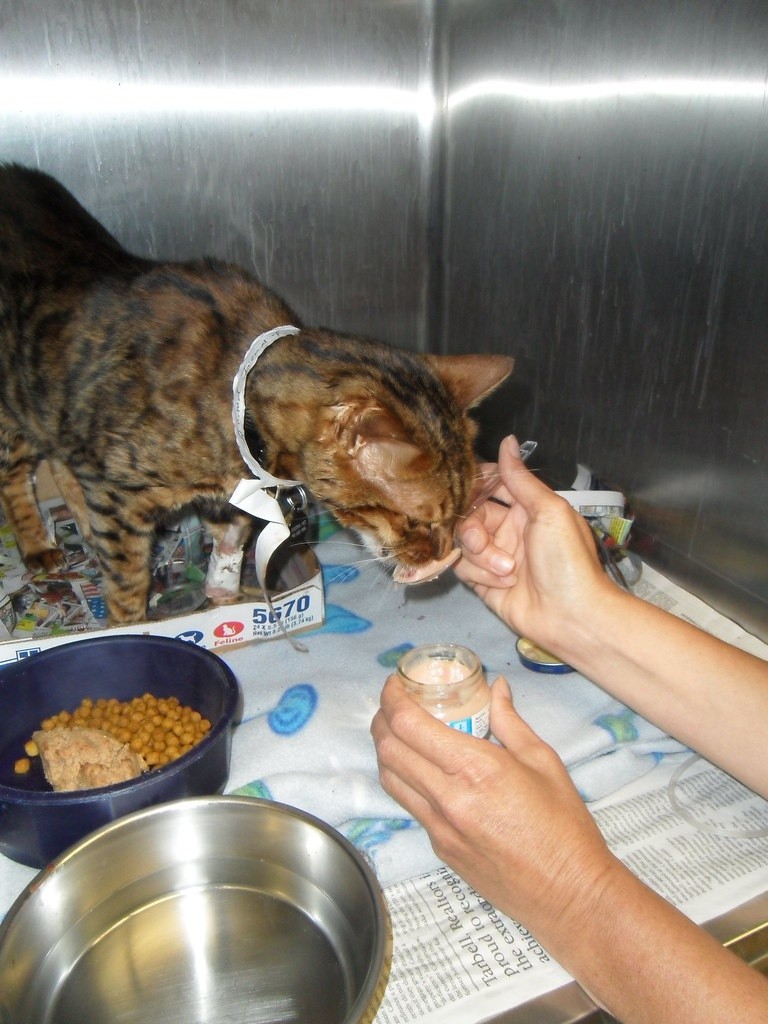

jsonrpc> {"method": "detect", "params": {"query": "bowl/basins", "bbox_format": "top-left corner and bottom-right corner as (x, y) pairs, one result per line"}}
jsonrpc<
(0, 631), (245, 871)
(0, 793), (393, 1024)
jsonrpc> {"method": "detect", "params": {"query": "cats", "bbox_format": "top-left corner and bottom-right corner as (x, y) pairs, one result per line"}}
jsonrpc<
(0, 158), (519, 630)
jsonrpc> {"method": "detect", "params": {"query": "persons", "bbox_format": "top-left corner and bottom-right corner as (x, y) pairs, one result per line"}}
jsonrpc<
(372, 433), (767, 1024)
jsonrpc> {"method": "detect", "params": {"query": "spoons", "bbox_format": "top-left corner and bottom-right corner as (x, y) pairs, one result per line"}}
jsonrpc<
(389, 439), (539, 585)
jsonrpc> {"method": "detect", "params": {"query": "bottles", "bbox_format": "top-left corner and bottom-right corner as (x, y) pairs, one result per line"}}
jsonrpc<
(397, 637), (492, 749)
